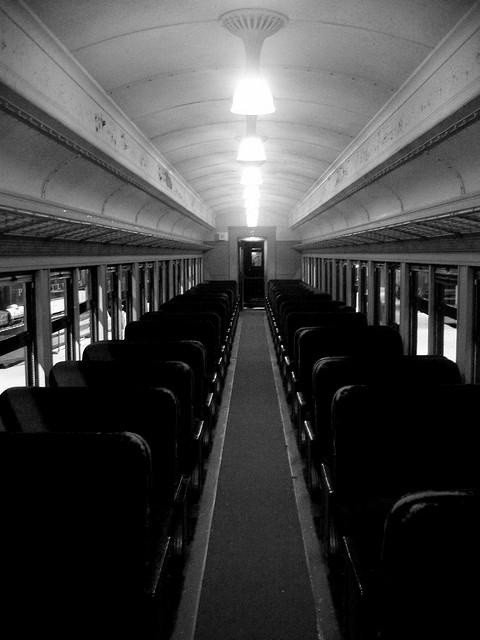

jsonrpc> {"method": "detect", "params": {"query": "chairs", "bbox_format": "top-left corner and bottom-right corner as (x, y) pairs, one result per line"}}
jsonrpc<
(265, 279), (369, 388)
(286, 322), (480, 502)
(3, 385), (214, 637)
(310, 380), (479, 635)
(124, 280), (237, 380)
(47, 339), (223, 429)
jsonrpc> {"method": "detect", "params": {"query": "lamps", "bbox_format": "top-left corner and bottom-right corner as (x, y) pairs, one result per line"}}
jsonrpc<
(218, 9), (288, 117)
(236, 116), (267, 229)
